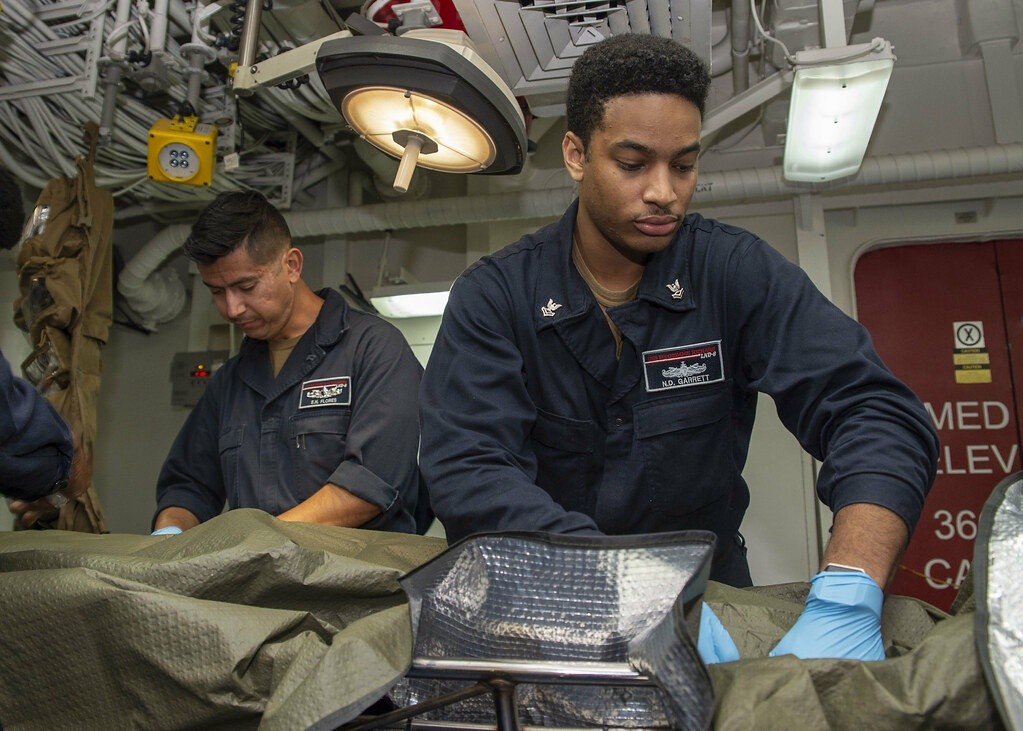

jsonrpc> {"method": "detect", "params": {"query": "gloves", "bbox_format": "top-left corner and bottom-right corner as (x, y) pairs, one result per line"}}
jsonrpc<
(768, 572), (885, 662)
(697, 600), (741, 665)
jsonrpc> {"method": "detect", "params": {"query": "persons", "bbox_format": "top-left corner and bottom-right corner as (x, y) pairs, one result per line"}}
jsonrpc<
(0, 348), (74, 527)
(149, 190), (436, 536)
(419, 34), (941, 665)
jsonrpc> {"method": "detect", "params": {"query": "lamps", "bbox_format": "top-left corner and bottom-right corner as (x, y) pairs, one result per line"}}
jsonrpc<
(781, 38), (898, 183)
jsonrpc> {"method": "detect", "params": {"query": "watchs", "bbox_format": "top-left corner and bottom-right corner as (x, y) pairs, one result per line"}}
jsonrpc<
(809, 562), (884, 619)
